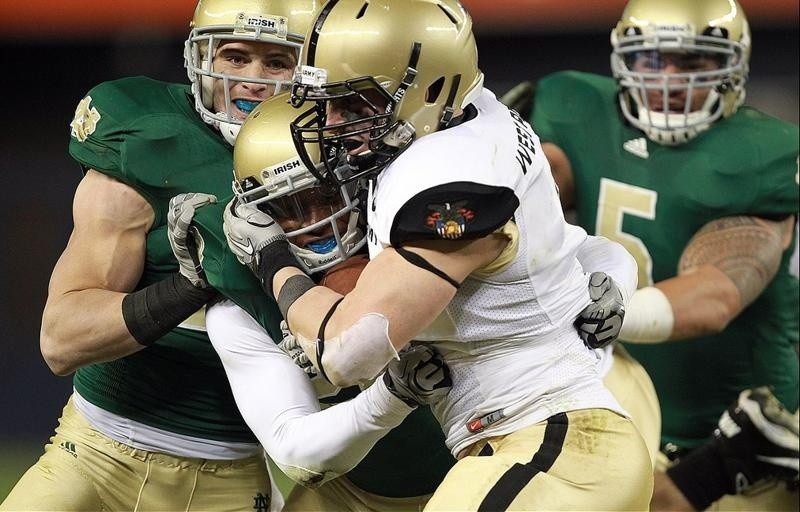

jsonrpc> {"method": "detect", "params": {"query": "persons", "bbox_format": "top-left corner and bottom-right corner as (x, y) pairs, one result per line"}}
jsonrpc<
(523, 0), (800, 512)
(0, 1), (322, 510)
(220, 0), (799, 511)
(203, 88), (640, 511)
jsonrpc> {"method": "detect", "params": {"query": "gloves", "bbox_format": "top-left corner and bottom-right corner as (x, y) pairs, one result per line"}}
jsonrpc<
(275, 319), (319, 376)
(572, 270), (626, 350)
(381, 340), (457, 410)
(222, 195), (288, 279)
(166, 192), (218, 290)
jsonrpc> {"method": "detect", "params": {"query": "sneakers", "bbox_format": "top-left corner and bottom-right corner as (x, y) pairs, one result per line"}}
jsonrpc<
(713, 385), (799, 488)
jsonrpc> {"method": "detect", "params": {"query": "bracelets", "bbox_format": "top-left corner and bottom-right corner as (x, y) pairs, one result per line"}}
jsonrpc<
(276, 274), (321, 333)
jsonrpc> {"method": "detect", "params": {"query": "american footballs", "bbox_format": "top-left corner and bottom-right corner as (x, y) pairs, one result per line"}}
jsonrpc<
(315, 254), (370, 293)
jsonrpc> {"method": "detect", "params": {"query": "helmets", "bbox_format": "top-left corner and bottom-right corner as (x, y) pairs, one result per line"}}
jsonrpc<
(190, 2), (326, 150)
(607, 1), (753, 146)
(292, 2), (480, 153)
(231, 91), (371, 277)
